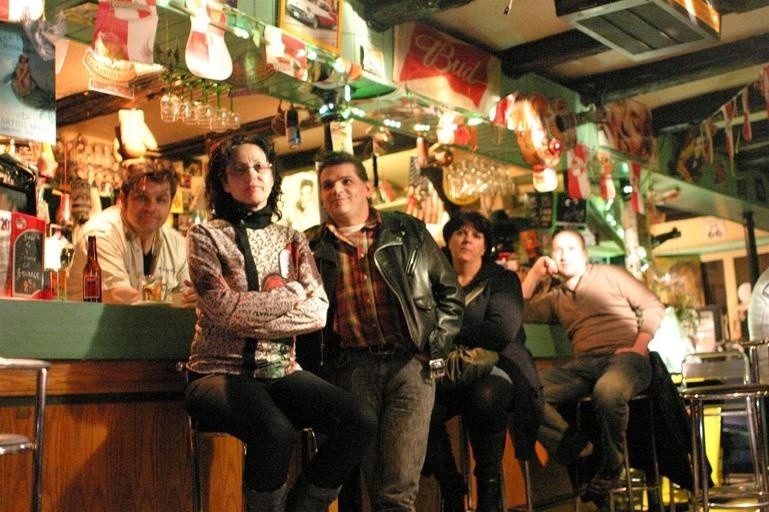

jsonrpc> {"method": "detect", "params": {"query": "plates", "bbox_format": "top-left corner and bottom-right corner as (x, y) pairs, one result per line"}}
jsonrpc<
(131, 302), (175, 306)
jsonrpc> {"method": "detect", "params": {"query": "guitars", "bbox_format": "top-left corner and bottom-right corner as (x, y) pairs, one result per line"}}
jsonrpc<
(512, 93), (606, 166)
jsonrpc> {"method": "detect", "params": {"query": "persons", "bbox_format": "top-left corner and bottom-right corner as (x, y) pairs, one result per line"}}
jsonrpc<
(521, 227), (666, 496)
(184, 132), (379, 512)
(15, 53), (31, 96)
(67, 159), (198, 309)
(295, 151), (465, 512)
(427, 211), (544, 512)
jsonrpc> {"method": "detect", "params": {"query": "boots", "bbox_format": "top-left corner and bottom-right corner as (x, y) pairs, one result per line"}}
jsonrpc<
(293, 478), (342, 512)
(243, 473), (289, 512)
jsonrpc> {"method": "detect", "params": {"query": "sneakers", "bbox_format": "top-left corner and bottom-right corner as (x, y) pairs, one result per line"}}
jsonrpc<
(589, 457), (622, 495)
(575, 443), (600, 495)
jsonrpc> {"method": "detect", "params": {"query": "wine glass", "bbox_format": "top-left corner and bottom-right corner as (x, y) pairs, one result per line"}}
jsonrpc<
(160, 75), (240, 134)
(446, 158), (515, 199)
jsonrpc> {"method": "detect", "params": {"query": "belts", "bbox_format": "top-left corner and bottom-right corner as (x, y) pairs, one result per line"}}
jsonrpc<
(351, 343), (398, 357)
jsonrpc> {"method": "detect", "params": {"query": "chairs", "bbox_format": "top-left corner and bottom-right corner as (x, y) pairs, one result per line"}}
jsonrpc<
(0, 355), (53, 512)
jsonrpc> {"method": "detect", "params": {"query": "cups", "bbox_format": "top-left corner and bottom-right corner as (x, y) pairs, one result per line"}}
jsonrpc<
(137, 273), (169, 301)
(49, 271), (67, 300)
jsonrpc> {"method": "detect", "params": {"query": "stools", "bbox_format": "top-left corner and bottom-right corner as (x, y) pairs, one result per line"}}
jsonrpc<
(175, 343), (318, 512)
(574, 390), (664, 511)
(437, 394), (534, 511)
(624, 345), (769, 511)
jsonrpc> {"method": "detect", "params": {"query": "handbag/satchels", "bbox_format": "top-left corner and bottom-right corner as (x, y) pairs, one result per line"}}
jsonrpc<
(439, 345), (498, 387)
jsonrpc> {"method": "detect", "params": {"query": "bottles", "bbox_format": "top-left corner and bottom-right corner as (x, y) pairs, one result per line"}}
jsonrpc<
(81, 234), (102, 302)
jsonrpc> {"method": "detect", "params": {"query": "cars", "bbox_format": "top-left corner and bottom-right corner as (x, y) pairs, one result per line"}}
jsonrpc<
(287, 0), (336, 31)
